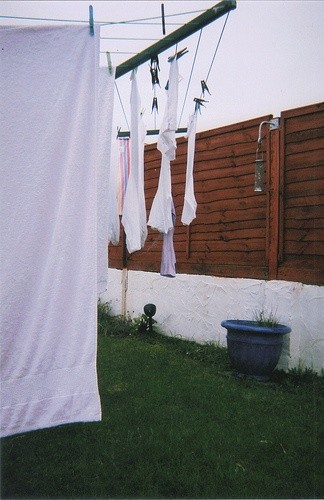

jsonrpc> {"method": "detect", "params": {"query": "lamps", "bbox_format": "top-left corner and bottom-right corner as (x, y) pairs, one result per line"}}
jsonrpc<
(253, 119), (279, 191)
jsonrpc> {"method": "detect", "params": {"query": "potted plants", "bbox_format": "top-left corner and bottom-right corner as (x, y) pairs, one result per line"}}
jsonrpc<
(221, 303), (291, 371)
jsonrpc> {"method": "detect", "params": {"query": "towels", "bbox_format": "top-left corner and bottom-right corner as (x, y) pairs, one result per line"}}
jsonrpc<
(0, 24), (103, 440)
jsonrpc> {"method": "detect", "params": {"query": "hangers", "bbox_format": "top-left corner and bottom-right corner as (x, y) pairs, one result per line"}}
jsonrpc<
(115, 0), (237, 140)
